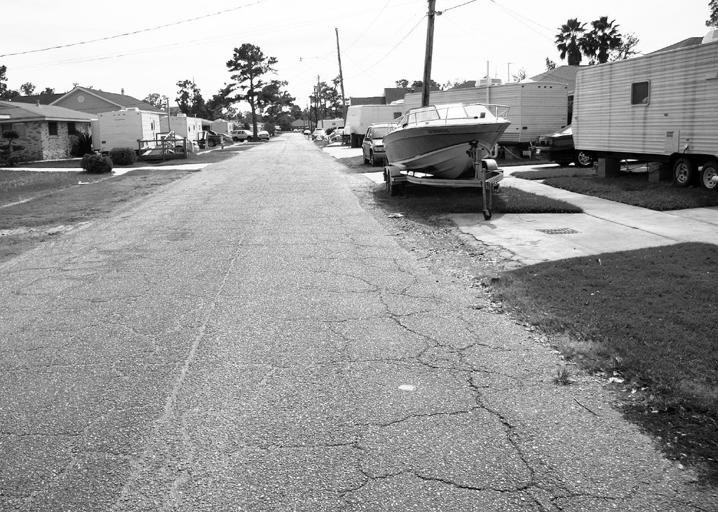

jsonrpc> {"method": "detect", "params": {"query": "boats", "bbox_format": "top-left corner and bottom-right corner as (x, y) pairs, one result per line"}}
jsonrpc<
(382, 102), (512, 178)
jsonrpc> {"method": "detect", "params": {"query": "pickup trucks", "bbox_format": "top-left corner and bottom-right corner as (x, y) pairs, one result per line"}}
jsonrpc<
(202, 130), (223, 147)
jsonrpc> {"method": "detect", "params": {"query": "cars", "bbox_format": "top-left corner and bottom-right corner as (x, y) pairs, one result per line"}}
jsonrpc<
(312, 128), (326, 140)
(304, 130), (311, 135)
(362, 122), (399, 167)
(217, 129), (270, 142)
(161, 134), (200, 153)
(533, 124), (594, 168)
